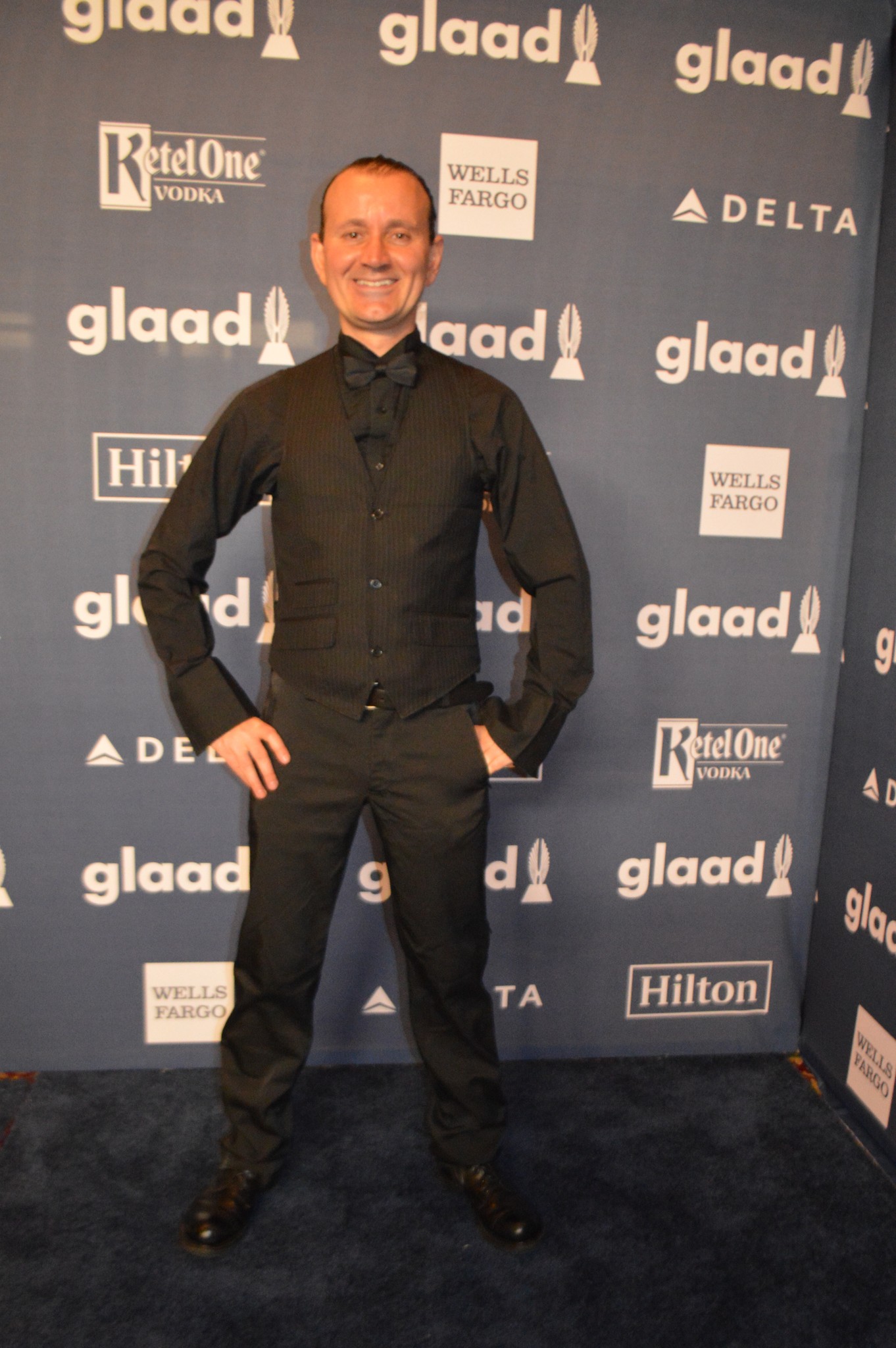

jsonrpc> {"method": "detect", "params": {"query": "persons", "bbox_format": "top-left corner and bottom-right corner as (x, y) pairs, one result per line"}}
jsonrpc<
(129, 152), (600, 1258)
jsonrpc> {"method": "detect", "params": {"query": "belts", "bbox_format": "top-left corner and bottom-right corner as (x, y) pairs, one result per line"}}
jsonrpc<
(365, 682), (497, 712)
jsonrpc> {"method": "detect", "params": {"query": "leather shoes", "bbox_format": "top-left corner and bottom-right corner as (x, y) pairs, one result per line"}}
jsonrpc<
(182, 1169), (268, 1256)
(463, 1163), (540, 1247)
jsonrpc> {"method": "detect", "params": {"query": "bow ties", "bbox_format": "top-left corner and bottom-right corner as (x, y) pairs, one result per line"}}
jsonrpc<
(344, 354), (420, 389)
